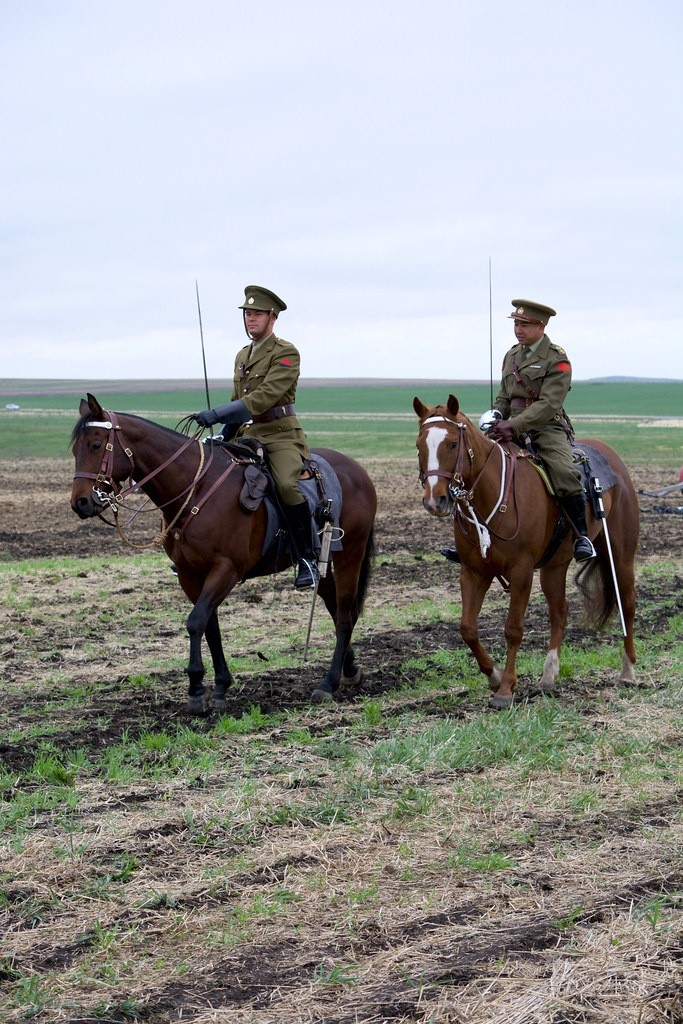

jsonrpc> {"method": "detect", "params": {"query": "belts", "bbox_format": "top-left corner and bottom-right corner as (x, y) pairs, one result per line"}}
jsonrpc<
(511, 398), (539, 407)
(251, 405), (297, 423)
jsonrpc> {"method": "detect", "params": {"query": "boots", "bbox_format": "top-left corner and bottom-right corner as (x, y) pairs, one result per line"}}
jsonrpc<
(440, 549), (461, 564)
(563, 492), (597, 563)
(286, 500), (320, 587)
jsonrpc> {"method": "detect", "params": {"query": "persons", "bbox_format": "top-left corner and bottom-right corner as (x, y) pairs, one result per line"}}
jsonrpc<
(439, 300), (593, 564)
(171, 285), (320, 586)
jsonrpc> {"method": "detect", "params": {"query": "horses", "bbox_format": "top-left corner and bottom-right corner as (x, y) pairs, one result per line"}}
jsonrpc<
(67, 391), (378, 715)
(412, 392), (641, 711)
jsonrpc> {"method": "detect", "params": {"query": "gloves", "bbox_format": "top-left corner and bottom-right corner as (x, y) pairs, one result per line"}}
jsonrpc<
(489, 419), (514, 438)
(217, 424), (242, 442)
(192, 399), (250, 428)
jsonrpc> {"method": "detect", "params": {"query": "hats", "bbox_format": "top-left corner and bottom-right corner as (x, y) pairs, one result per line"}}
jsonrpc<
(238, 285), (287, 340)
(506, 299), (557, 326)
